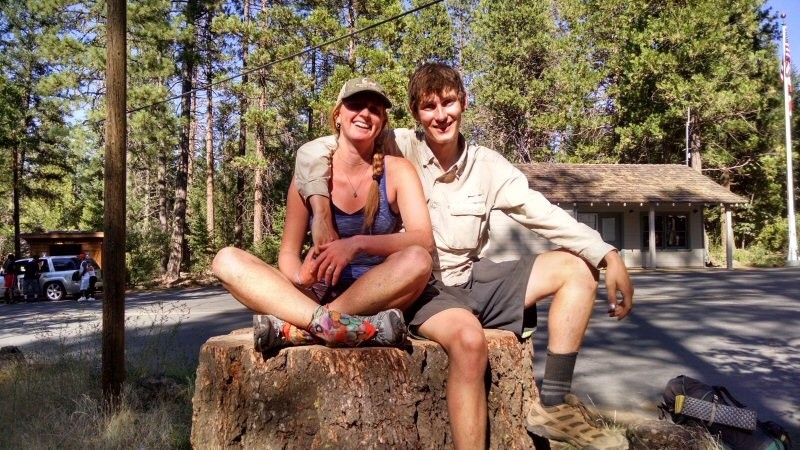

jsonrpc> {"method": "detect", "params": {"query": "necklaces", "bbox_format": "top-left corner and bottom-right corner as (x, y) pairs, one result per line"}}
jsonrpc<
(334, 146), (372, 198)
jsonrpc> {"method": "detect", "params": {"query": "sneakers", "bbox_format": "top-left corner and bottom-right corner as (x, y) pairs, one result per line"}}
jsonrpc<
(254, 313), (288, 352)
(353, 308), (404, 346)
(526, 393), (629, 450)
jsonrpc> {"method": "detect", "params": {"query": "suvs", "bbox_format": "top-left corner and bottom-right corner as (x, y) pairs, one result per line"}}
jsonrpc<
(0, 255), (104, 301)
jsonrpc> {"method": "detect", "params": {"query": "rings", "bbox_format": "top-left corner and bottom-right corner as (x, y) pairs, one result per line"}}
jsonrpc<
(328, 266), (334, 272)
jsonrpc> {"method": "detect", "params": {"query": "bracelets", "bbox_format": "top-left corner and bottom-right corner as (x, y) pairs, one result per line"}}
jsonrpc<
(297, 271), (312, 291)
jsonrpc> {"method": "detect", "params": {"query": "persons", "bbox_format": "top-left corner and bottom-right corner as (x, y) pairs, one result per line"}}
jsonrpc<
(293, 62), (634, 450)
(212, 78), (436, 353)
(3, 254), (97, 304)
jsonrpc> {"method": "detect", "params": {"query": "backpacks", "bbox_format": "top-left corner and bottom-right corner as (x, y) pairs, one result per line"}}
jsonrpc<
(658, 376), (792, 450)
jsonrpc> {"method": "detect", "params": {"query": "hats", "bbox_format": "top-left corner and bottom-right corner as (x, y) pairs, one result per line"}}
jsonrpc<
(337, 78), (392, 108)
(77, 254), (86, 258)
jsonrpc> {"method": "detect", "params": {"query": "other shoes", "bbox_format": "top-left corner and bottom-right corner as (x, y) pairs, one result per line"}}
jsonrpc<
(78, 296), (86, 301)
(88, 297), (95, 301)
